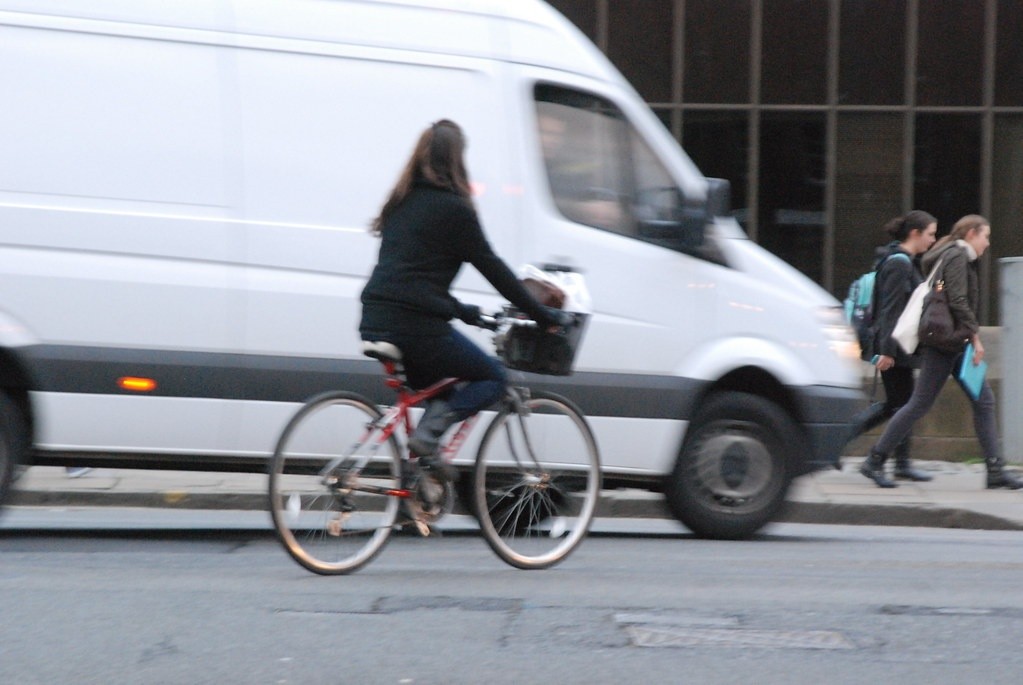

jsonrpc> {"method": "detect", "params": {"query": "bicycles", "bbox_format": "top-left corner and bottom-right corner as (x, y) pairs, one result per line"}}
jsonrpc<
(267, 309), (604, 576)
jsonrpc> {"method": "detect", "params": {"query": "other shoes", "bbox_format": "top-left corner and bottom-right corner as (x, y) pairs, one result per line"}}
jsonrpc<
(408, 438), (458, 482)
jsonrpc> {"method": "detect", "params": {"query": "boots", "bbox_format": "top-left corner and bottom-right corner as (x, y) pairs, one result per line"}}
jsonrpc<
(984, 455), (1023, 489)
(832, 402), (886, 470)
(892, 406), (934, 481)
(858, 446), (898, 490)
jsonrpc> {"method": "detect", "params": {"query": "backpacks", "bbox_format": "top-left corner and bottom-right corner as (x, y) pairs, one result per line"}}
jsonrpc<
(844, 254), (911, 362)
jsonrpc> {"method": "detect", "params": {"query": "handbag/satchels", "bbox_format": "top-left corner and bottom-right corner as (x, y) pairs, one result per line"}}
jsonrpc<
(917, 287), (955, 347)
(503, 278), (563, 368)
(891, 250), (949, 354)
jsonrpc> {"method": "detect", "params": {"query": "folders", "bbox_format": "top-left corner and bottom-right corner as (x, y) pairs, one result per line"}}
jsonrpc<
(959, 344), (988, 400)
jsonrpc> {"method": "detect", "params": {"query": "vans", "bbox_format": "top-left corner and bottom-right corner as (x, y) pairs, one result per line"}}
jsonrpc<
(0, 0), (871, 541)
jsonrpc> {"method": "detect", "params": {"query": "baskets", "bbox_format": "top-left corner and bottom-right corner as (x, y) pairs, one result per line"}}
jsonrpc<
(502, 304), (589, 376)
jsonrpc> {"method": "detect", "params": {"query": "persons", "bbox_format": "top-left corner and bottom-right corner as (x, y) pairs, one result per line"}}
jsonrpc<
(845, 211), (1023, 491)
(360, 120), (576, 480)
